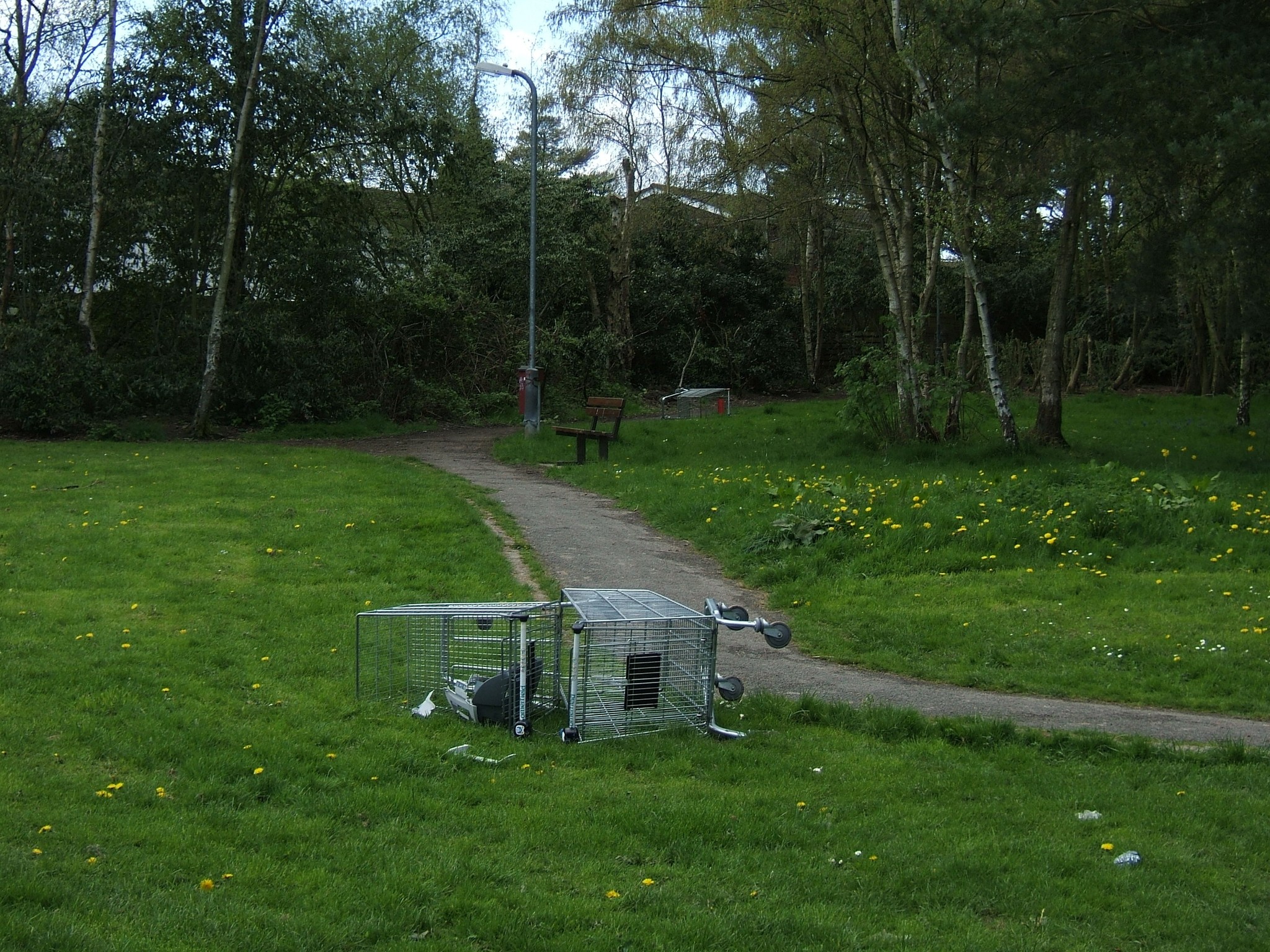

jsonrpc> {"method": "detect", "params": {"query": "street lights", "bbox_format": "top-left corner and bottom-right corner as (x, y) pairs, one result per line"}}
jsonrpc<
(475, 59), (539, 440)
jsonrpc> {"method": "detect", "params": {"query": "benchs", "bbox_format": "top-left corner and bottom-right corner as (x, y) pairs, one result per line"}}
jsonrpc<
(551, 395), (626, 467)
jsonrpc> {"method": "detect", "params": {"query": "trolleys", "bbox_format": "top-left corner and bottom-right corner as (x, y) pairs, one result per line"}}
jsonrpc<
(353, 599), (562, 739)
(556, 583), (793, 747)
(661, 387), (730, 418)
